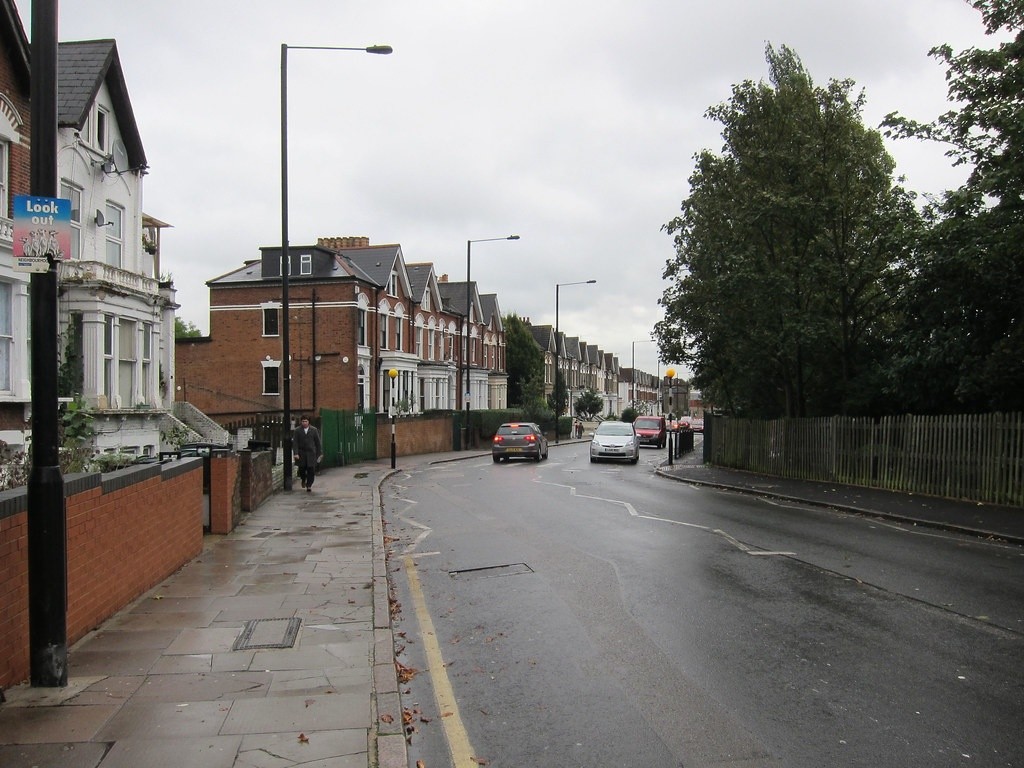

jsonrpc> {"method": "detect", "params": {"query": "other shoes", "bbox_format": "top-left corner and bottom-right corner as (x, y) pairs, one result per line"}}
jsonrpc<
(301, 481), (312, 494)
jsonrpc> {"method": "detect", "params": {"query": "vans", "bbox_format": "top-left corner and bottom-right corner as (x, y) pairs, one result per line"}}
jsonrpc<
(634, 416), (667, 448)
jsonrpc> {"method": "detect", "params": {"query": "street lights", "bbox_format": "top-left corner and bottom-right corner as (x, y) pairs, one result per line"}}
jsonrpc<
(555, 280), (596, 442)
(633, 340), (656, 409)
(666, 368), (675, 465)
(281, 43), (393, 492)
(465, 235), (521, 448)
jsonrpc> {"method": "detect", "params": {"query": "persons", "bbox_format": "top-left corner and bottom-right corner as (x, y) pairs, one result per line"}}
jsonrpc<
(577, 422), (584, 439)
(293, 415), (323, 493)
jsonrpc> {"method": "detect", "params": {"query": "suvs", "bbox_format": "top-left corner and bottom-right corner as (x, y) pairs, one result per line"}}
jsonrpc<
(492, 422), (549, 462)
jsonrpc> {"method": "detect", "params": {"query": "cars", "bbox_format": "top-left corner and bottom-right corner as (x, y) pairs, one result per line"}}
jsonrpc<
(665, 416), (704, 433)
(589, 421), (641, 463)
